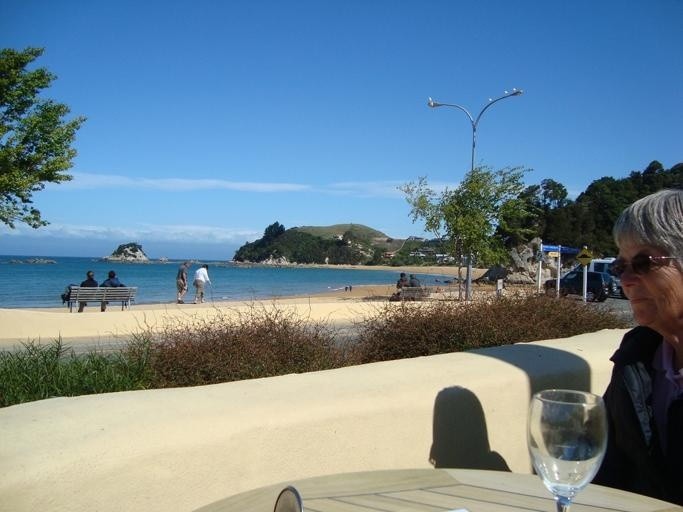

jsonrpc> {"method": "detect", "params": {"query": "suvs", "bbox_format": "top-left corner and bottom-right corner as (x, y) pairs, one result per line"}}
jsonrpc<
(546, 271), (617, 302)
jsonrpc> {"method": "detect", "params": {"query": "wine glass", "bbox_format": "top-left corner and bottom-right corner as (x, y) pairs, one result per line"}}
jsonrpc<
(524, 387), (610, 512)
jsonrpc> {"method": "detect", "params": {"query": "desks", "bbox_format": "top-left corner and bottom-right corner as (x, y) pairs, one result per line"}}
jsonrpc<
(187, 470), (678, 512)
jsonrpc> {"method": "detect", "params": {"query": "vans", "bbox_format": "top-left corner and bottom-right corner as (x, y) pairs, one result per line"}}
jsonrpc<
(563, 258), (621, 287)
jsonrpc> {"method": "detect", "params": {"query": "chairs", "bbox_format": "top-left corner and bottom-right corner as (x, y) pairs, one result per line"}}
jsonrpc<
(67, 286), (138, 312)
(389, 286), (431, 302)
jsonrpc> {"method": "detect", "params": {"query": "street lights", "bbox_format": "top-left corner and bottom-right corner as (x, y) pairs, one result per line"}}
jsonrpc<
(429, 88), (524, 300)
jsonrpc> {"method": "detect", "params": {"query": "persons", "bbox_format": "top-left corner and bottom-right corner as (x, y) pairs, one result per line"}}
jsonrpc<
(408, 273), (421, 289)
(79, 270), (98, 308)
(395, 273), (408, 296)
(549, 186), (681, 511)
(175, 259), (193, 304)
(191, 263), (211, 305)
(98, 270), (128, 305)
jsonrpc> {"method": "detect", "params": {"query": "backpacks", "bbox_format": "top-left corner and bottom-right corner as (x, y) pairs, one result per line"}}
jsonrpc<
(61, 284), (79, 301)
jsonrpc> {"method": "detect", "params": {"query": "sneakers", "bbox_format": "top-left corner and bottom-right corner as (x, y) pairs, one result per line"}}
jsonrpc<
(78, 301), (109, 313)
(176, 297), (206, 304)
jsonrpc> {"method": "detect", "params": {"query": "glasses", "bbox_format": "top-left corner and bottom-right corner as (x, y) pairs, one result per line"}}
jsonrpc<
(607, 254), (683, 277)
